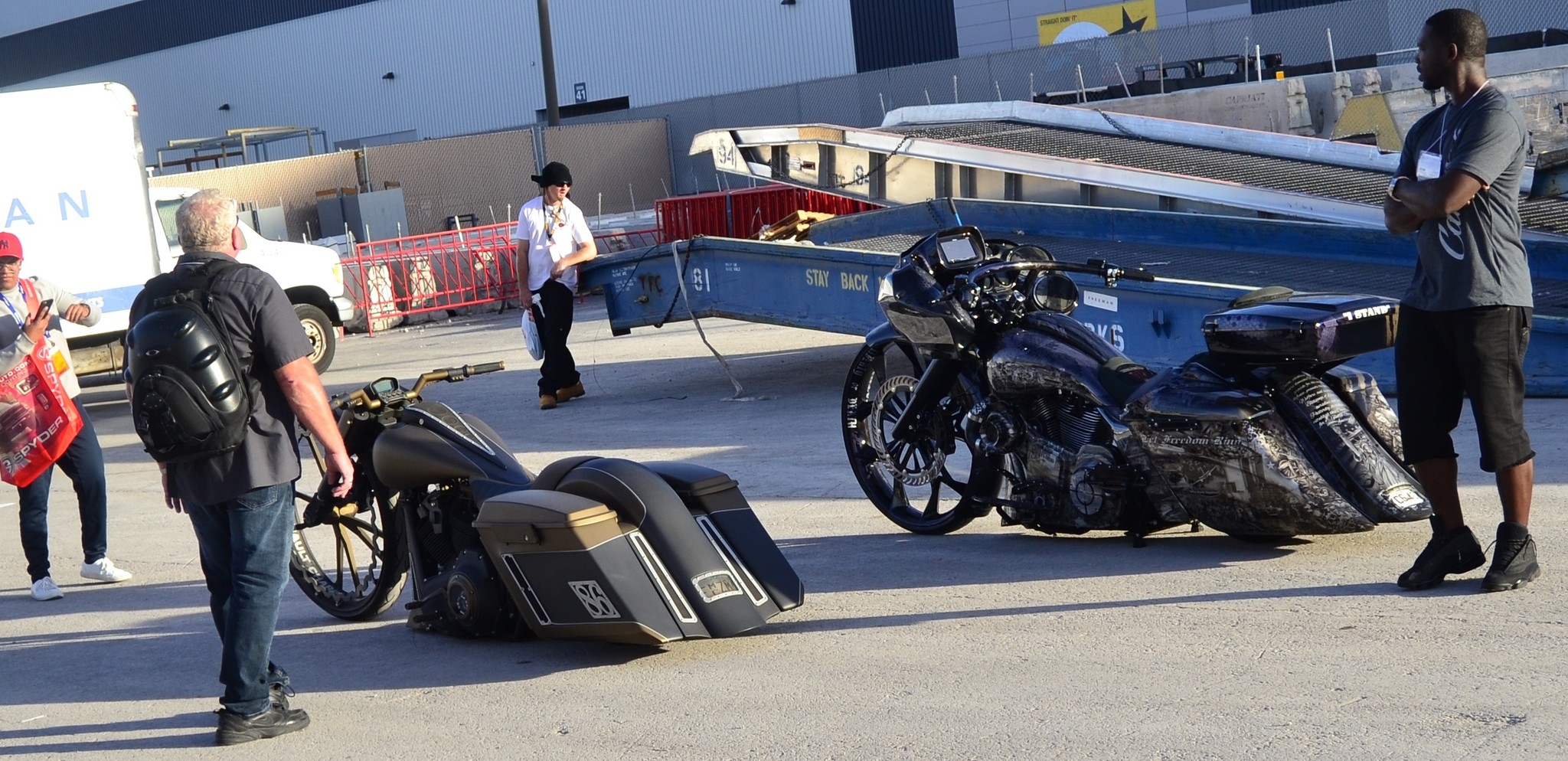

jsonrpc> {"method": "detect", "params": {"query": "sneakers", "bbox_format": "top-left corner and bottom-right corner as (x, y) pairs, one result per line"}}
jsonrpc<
(31, 575), (64, 602)
(215, 703), (311, 746)
(540, 393), (557, 410)
(80, 557), (133, 583)
(1478, 521), (1542, 592)
(1396, 514), (1489, 591)
(269, 683), (290, 712)
(556, 380), (586, 403)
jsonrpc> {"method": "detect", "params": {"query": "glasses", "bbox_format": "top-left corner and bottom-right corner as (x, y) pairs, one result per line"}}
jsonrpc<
(553, 180), (572, 187)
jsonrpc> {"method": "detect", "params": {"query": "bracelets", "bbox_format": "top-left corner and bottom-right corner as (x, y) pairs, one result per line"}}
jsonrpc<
(80, 303), (90, 317)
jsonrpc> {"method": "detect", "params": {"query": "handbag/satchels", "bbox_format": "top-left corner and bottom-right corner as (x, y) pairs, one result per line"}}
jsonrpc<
(521, 307), (544, 361)
(0, 338), (84, 489)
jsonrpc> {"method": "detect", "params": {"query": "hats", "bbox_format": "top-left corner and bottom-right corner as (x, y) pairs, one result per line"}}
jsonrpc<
(542, 161), (573, 186)
(0, 231), (24, 262)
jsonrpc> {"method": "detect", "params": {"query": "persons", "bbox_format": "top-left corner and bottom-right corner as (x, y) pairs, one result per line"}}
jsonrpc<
(1382, 7), (1542, 594)
(516, 162), (597, 409)
(122, 188), (355, 745)
(0, 231), (131, 600)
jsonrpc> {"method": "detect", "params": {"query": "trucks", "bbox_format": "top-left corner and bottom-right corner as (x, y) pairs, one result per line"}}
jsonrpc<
(0, 80), (345, 376)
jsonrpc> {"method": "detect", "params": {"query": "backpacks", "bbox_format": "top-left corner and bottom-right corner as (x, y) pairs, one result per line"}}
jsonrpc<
(115, 258), (265, 466)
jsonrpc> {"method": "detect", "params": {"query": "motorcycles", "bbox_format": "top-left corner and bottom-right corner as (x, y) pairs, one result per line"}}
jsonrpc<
(839, 195), (1434, 537)
(290, 359), (805, 647)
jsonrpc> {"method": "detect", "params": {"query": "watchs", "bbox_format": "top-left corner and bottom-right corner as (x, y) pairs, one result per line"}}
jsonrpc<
(1387, 176), (1410, 202)
